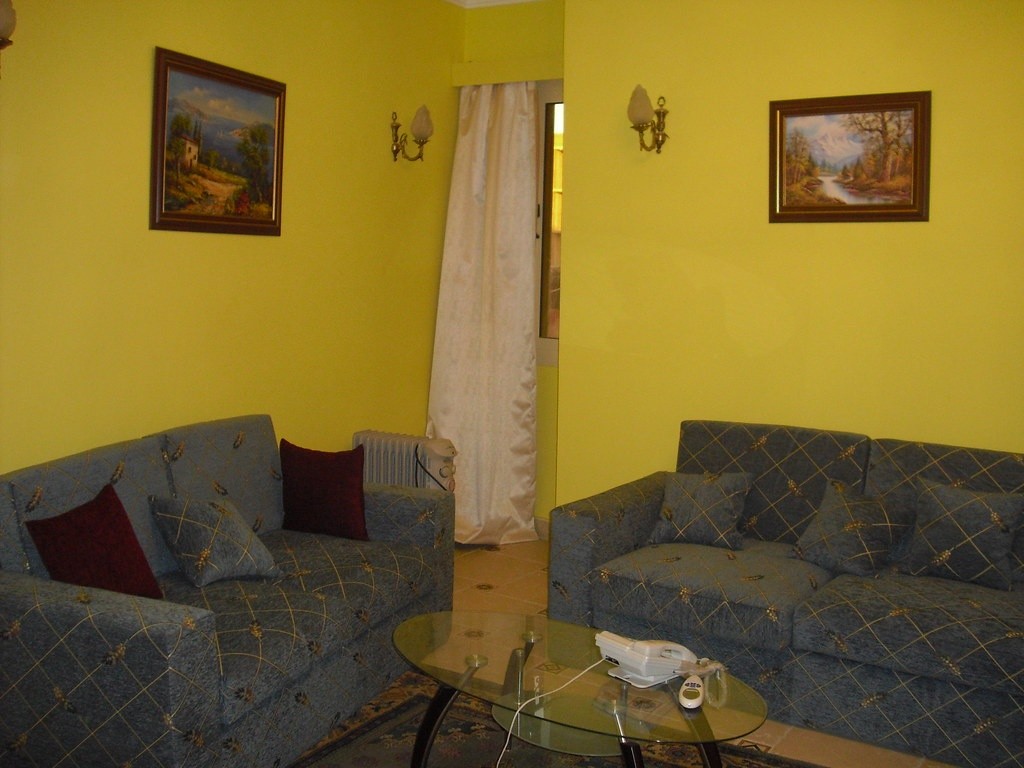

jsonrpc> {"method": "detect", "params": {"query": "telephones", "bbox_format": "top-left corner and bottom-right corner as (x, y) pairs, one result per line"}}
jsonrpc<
(595, 631), (697, 689)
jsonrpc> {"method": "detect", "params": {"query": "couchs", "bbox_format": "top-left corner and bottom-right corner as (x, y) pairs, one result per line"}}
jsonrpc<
(0, 412), (457, 766)
(547, 420), (1024, 768)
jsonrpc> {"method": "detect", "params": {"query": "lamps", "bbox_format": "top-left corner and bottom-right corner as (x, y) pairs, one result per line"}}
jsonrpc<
(389, 101), (436, 162)
(626, 82), (669, 155)
(1, 0), (17, 51)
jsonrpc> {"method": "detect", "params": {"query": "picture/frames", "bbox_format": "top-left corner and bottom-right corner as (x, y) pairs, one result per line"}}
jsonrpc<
(766, 89), (932, 224)
(150, 44), (286, 239)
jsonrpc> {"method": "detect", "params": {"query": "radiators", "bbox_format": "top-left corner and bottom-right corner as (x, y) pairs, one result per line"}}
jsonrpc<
(352, 429), (458, 492)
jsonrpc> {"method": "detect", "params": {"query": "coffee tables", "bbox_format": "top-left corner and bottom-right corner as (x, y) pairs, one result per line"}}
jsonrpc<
(389, 610), (768, 766)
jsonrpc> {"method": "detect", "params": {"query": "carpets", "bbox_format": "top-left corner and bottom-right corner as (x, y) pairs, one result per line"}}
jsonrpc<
(284, 664), (823, 767)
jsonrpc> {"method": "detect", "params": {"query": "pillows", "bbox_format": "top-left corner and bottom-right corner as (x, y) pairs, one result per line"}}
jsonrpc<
(150, 497), (287, 586)
(899, 475), (1024, 592)
(785, 479), (915, 578)
(648, 470), (754, 552)
(23, 484), (164, 601)
(280, 437), (371, 542)
